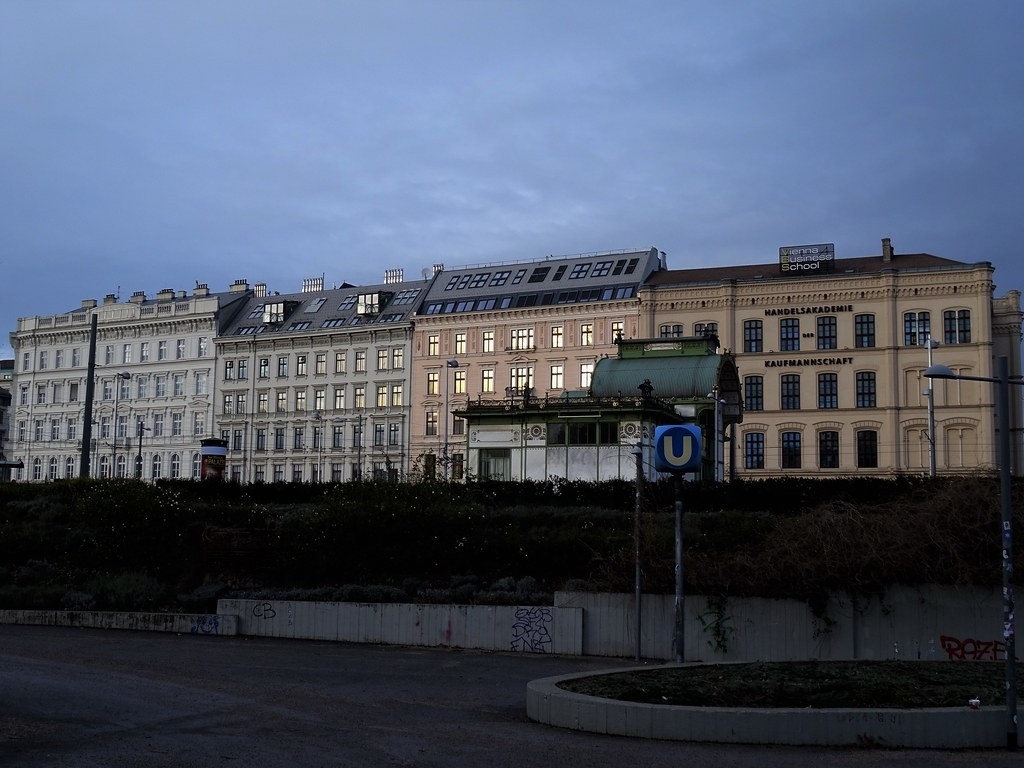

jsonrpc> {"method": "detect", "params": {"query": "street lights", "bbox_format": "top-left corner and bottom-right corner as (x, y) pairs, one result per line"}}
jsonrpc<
(923, 355), (1018, 752)
(444, 359), (459, 484)
(136, 420), (152, 479)
(112, 370), (131, 478)
